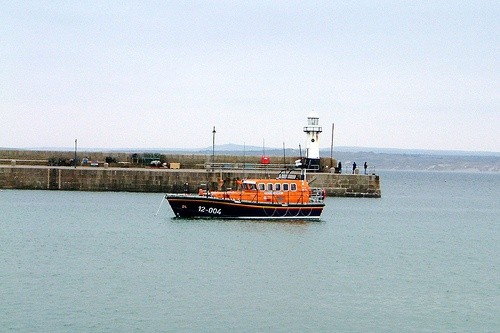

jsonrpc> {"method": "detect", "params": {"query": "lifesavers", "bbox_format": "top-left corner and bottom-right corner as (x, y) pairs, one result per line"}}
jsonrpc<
(261, 156), (271, 164)
(322, 188), (327, 200)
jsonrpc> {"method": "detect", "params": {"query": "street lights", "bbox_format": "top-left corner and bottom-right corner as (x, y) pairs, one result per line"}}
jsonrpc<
(212, 125), (217, 164)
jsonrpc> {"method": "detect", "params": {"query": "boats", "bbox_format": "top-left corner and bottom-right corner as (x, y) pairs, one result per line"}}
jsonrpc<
(164, 164), (327, 222)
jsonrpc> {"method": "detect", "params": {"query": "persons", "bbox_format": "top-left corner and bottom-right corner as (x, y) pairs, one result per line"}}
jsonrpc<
(364, 162), (368, 174)
(352, 162), (356, 174)
